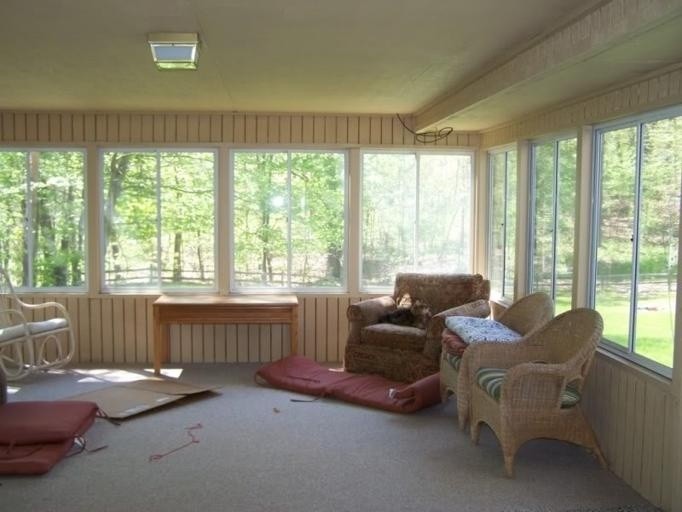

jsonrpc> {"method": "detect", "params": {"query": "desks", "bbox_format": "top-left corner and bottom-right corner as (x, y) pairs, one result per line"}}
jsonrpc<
(151, 293), (300, 376)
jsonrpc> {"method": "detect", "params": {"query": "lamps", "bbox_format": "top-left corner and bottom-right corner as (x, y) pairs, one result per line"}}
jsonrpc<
(146, 30), (203, 70)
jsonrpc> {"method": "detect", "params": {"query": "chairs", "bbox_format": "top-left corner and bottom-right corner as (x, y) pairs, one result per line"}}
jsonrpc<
(0, 263), (76, 381)
(467, 305), (607, 475)
(437, 292), (556, 430)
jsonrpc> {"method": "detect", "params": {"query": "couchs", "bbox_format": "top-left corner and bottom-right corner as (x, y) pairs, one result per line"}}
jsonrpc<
(344, 271), (491, 382)
